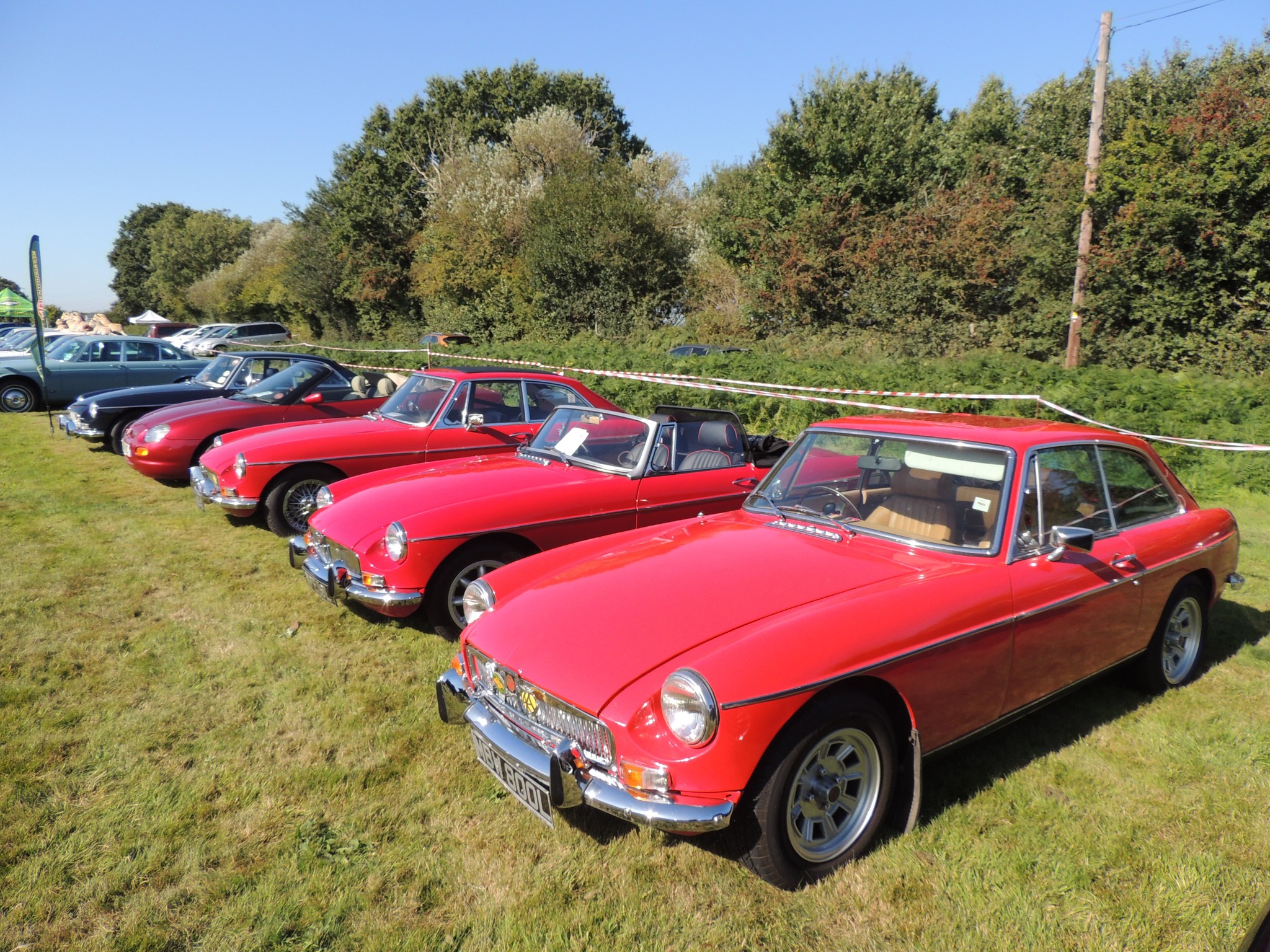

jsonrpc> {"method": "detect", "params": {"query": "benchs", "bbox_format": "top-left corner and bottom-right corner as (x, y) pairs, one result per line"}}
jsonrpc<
(956, 486), (1127, 544)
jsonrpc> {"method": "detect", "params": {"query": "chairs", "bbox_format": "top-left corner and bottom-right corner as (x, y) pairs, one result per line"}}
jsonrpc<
(343, 375), (393, 402)
(98, 348), (110, 361)
(865, 459), (1084, 545)
(622, 414), (739, 470)
(266, 368), (278, 378)
(484, 409), (504, 423)
(289, 370), (304, 378)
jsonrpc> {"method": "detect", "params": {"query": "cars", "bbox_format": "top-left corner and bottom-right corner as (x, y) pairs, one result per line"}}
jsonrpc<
(666, 346), (747, 356)
(0, 324), (250, 414)
(433, 415), (1243, 893)
(120, 364), (506, 485)
(188, 364), (649, 541)
(56, 350), (372, 456)
(286, 401), (871, 644)
(160, 323), (238, 357)
(418, 332), (477, 350)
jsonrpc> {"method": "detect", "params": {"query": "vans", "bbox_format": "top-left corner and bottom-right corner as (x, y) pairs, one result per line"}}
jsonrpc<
(147, 324), (199, 339)
(193, 323), (292, 357)
